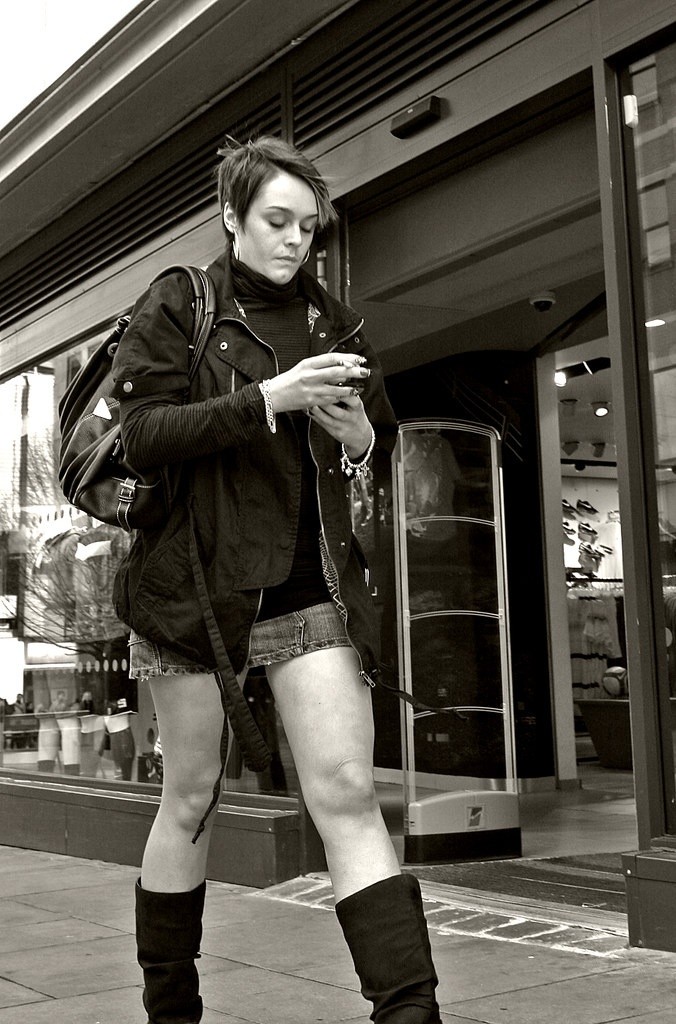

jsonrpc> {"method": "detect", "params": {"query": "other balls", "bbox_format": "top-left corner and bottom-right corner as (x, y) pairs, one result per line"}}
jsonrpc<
(601, 665), (628, 697)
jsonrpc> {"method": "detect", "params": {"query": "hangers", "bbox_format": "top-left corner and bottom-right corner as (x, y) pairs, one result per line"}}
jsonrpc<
(568, 575), (676, 593)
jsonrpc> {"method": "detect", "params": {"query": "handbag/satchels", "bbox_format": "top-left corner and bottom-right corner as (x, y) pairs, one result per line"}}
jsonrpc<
(58, 264), (215, 529)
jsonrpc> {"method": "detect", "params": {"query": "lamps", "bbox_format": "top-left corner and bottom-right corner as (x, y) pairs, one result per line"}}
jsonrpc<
(583, 356), (607, 375)
(591, 442), (607, 458)
(561, 441), (579, 457)
(574, 464), (586, 471)
(563, 402), (576, 418)
(591, 401), (609, 418)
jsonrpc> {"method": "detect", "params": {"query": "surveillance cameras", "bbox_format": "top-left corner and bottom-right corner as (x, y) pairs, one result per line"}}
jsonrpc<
(531, 290), (558, 313)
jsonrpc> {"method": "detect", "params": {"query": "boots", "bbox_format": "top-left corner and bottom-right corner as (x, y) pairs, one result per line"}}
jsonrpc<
(335, 873), (442, 1024)
(135, 876), (206, 1024)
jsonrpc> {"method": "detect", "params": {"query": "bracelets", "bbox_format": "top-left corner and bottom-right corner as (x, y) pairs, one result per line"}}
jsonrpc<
(263, 379), (276, 433)
(340, 419), (376, 480)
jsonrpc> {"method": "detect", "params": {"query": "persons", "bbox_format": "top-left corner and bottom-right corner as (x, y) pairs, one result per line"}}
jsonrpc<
(24, 517), (139, 781)
(113, 137), (447, 1024)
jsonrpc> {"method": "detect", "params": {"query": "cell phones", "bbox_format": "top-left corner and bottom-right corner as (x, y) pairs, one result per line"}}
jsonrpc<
(309, 380), (365, 416)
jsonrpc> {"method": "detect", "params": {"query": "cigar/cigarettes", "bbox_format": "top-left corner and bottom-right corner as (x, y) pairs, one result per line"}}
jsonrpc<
(337, 360), (356, 367)
